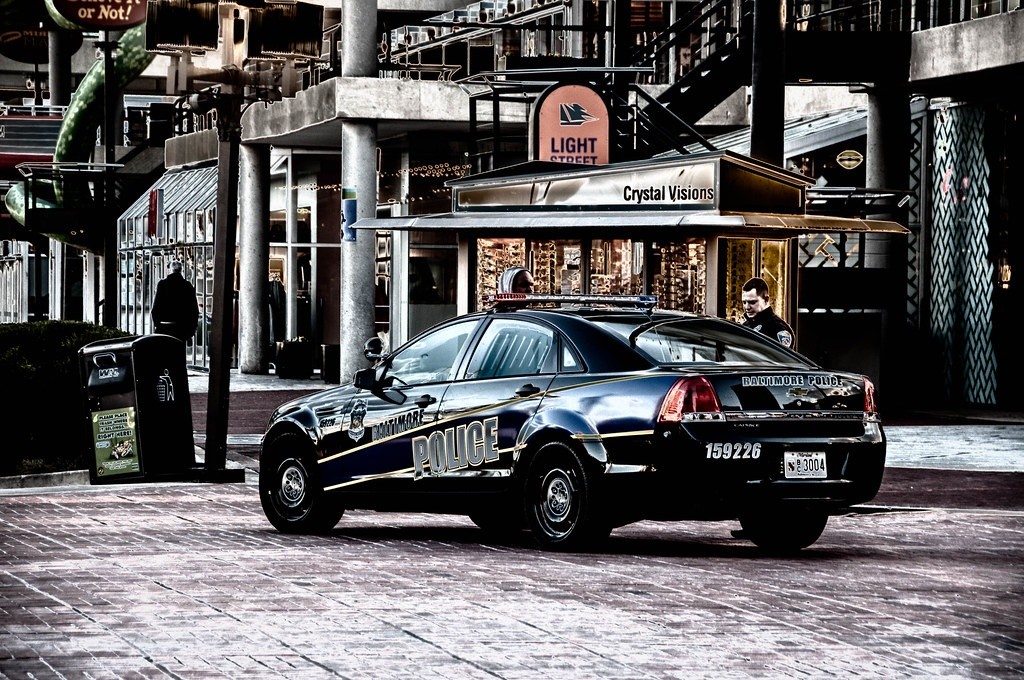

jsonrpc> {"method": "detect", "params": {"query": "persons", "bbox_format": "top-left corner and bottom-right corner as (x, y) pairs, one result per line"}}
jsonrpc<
(151, 260), (199, 348)
(498, 267), (534, 310)
(730, 278), (796, 540)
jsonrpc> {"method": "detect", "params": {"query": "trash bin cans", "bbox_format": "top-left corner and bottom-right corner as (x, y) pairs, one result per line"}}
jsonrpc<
(78, 334), (196, 486)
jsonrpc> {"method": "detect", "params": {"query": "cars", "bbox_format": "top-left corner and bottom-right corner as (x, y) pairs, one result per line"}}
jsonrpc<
(258, 294), (887, 551)
